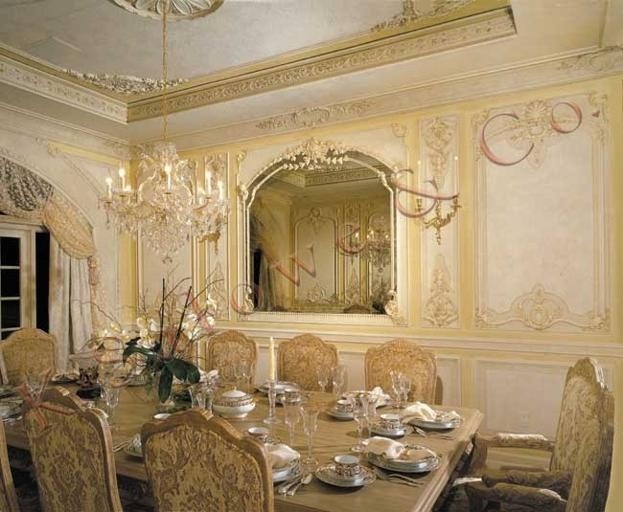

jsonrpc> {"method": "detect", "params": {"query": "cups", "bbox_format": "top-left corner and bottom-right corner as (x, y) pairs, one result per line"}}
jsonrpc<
(186, 385), (213, 411)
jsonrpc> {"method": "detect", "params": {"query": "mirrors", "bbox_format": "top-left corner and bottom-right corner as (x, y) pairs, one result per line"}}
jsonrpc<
(236, 137), (401, 321)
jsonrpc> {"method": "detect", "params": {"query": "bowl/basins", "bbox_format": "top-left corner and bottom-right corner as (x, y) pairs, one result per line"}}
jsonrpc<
(212, 384), (254, 420)
(173, 382), (210, 401)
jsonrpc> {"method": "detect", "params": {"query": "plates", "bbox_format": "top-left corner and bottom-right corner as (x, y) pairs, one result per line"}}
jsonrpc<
(153, 413), (169, 420)
(122, 446), (142, 458)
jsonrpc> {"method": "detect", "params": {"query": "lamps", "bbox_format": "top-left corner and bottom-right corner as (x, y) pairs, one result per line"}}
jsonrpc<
(335, 219), (391, 273)
(98, 0), (233, 258)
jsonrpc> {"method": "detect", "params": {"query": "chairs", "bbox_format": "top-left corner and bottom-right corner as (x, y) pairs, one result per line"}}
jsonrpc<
(1, 328), (59, 390)
(1, 385), (123, 511)
(365, 338), (436, 405)
(207, 329), (257, 384)
(141, 407), (274, 512)
(276, 334), (338, 392)
(465, 357), (614, 512)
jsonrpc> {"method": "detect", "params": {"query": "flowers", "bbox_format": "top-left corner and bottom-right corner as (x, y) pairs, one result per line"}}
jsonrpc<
(72, 270), (230, 400)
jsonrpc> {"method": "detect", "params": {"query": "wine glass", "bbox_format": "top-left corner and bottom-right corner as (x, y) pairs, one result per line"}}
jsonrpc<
(252, 363), (460, 499)
(0, 353), (124, 433)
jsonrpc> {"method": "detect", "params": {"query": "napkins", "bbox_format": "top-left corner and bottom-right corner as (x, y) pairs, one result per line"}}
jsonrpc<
(367, 386), (390, 408)
(402, 402), (464, 426)
(362, 436), (435, 462)
(264, 442), (301, 468)
